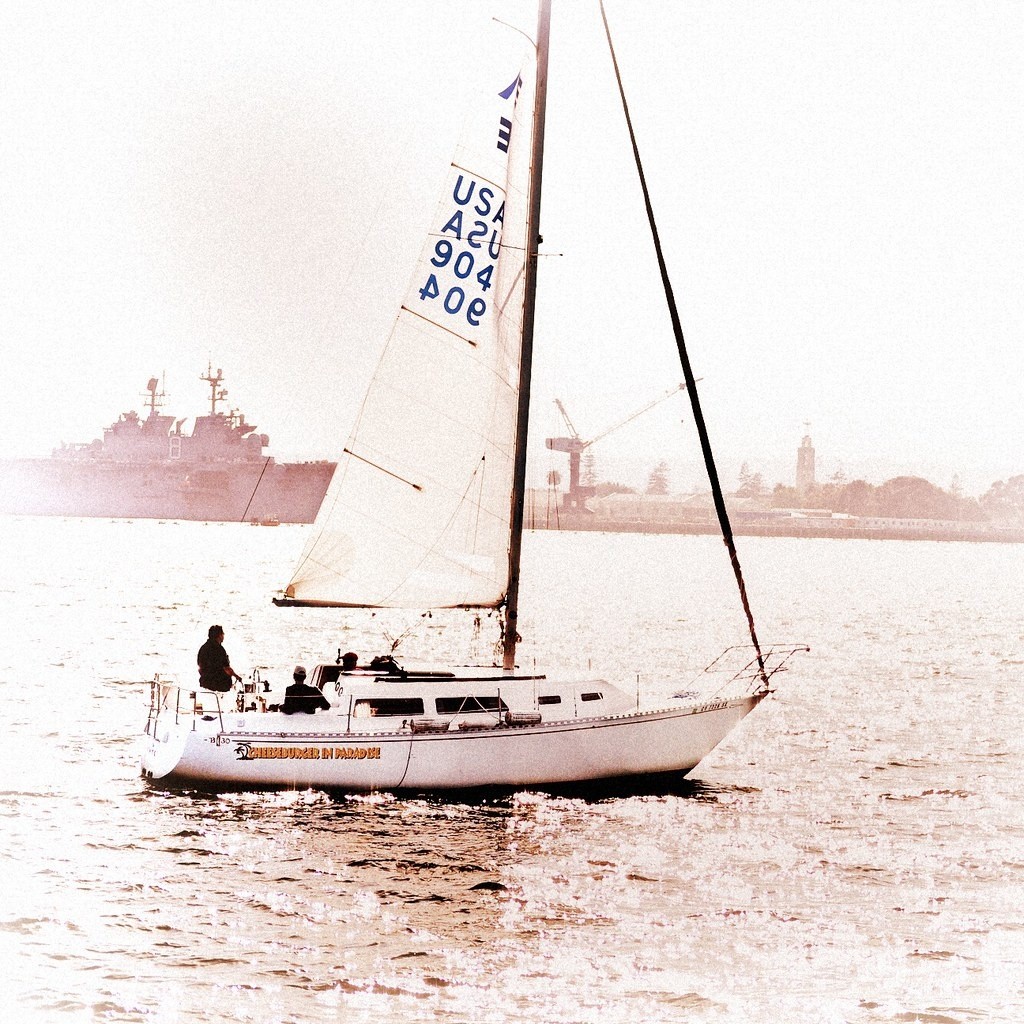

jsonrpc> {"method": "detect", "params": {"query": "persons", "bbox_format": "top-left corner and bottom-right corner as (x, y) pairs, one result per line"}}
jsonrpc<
(197, 625), (243, 713)
(280, 665), (330, 715)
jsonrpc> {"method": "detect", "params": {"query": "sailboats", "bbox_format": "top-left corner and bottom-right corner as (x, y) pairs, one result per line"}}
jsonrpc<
(131, 1), (814, 792)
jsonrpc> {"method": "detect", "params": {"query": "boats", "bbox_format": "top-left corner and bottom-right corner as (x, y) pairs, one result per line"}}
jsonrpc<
(0, 362), (339, 525)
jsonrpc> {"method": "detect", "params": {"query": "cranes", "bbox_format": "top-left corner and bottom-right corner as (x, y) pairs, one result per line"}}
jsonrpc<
(545, 377), (706, 514)
